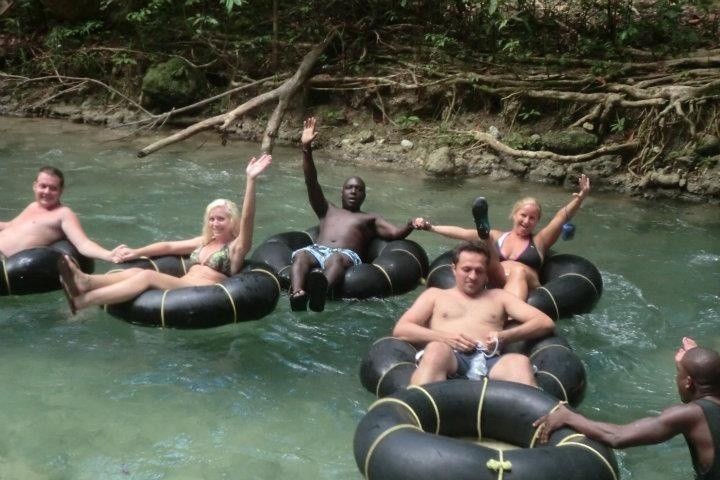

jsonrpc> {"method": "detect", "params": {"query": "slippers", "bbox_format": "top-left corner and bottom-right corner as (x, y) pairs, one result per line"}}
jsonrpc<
(57, 253), (82, 298)
(290, 289), (309, 312)
(59, 275), (78, 315)
(308, 269), (328, 313)
(472, 196), (491, 240)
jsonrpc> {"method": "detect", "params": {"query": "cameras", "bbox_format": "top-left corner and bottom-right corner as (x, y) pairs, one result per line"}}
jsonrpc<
(562, 223), (575, 241)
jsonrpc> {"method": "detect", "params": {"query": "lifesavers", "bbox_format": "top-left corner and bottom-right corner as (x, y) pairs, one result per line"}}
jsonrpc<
(0, 237), (93, 295)
(250, 226), (428, 299)
(427, 243), (601, 319)
(361, 332), (585, 407)
(102, 255), (280, 328)
(353, 379), (620, 480)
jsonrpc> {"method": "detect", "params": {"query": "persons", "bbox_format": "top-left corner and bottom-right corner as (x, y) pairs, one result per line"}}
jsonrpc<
(289, 116), (433, 313)
(531, 345), (720, 480)
(414, 173), (590, 321)
(0, 166), (135, 264)
(55, 152), (274, 315)
(675, 336), (698, 363)
(393, 241), (557, 392)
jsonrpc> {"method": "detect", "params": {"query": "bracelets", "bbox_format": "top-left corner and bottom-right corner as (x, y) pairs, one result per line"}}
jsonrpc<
(302, 147), (312, 154)
